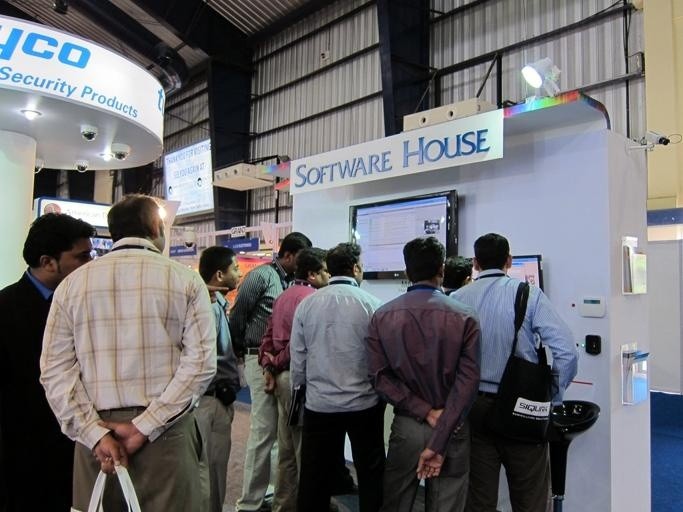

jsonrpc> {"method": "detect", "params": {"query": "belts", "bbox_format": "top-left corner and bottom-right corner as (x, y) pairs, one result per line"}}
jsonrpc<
(203, 385), (216, 398)
(245, 348), (260, 356)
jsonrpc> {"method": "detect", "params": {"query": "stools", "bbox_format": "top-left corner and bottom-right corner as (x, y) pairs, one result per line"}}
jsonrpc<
(546, 398), (601, 511)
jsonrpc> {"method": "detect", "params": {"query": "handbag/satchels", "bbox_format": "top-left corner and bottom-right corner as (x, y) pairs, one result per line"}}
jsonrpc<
(493, 353), (552, 447)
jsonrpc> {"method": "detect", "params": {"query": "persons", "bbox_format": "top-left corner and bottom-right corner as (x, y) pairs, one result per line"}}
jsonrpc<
(439, 255), (472, 299)
(37, 193), (220, 511)
(445, 232), (579, 511)
(194, 244), (242, 511)
(226, 232), (313, 511)
(365, 234), (483, 511)
(286, 240), (391, 511)
(256, 247), (330, 511)
(0, 214), (230, 511)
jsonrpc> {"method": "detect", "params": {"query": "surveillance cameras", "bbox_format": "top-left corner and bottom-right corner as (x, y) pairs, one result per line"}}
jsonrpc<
(646, 130), (670, 146)
(81, 127), (97, 141)
(35, 158), (43, 173)
(111, 143), (130, 161)
(321, 52), (328, 60)
(75, 160), (88, 173)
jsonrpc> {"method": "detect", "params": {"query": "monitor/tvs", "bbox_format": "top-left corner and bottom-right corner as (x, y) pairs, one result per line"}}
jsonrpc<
(348, 190), (459, 280)
(89, 235), (113, 259)
(472, 255), (544, 292)
(161, 136), (215, 224)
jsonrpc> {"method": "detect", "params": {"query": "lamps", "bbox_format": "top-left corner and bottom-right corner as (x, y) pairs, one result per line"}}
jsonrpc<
(520, 57), (561, 97)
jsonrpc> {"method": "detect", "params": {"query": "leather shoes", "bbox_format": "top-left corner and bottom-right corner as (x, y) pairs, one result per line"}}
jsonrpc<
(235, 501), (272, 511)
(332, 485), (358, 496)
